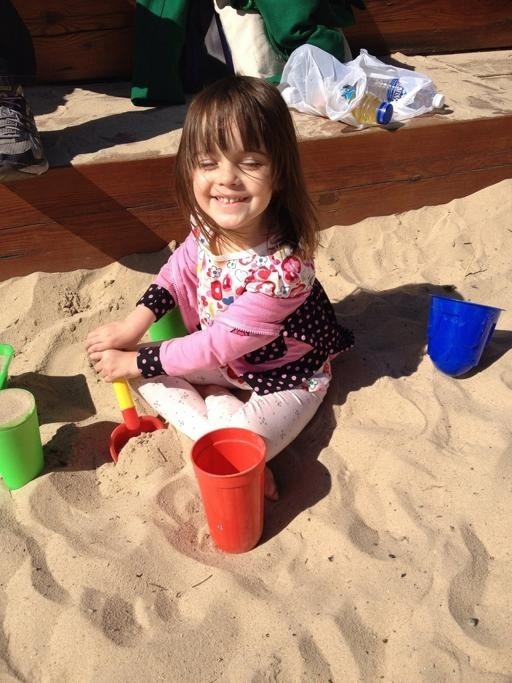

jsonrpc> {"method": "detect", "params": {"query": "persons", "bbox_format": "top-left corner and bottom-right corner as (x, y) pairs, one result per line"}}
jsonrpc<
(83, 77), (355, 500)
(0, 0), (47, 167)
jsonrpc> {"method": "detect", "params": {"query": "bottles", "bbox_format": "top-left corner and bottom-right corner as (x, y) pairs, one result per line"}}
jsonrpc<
(339, 84), (393, 126)
(364, 77), (445, 109)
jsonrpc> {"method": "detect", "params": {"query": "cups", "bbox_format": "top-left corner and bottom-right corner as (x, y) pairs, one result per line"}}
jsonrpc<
(426, 293), (506, 377)
(0, 387), (46, 490)
(147, 306), (189, 344)
(191, 428), (267, 552)
